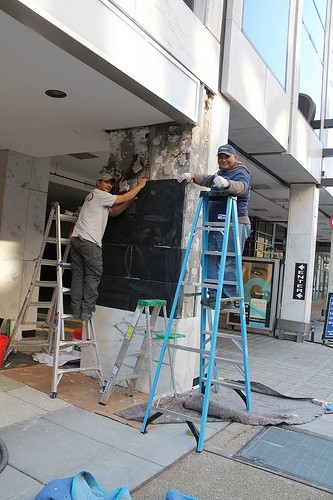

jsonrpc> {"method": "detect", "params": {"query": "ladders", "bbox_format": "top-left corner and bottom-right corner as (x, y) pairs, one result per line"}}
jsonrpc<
(3, 201), (107, 399)
(96, 298), (179, 408)
(140, 188), (253, 453)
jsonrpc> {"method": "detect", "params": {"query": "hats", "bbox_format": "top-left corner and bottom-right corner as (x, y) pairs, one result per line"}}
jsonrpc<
(97, 171), (116, 183)
(217, 144), (236, 156)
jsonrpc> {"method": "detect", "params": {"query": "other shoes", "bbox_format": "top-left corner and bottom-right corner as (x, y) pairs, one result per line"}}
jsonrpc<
(73, 314), (81, 320)
(81, 313), (92, 320)
(200, 297), (236, 310)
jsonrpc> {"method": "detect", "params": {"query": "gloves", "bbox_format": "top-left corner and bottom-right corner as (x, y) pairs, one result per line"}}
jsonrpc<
(212, 176), (230, 190)
(176, 172), (192, 184)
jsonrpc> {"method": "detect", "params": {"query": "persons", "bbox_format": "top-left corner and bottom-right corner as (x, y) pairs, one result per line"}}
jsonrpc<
(70, 172), (147, 320)
(177, 144), (251, 311)
(242, 261), (273, 299)
